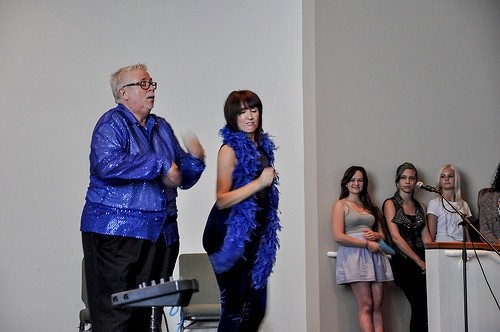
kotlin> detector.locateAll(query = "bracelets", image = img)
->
[366,240,368,248]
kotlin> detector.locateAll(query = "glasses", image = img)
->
[122,81,157,90]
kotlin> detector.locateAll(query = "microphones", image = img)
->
[416,181,436,192]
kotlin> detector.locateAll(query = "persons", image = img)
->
[479,163,500,245]
[202,90,279,332]
[382,162,433,332]
[427,164,472,242]
[333,166,394,332]
[80,65,205,332]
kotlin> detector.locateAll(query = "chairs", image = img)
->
[79,257,91,332]
[179,253,246,332]
